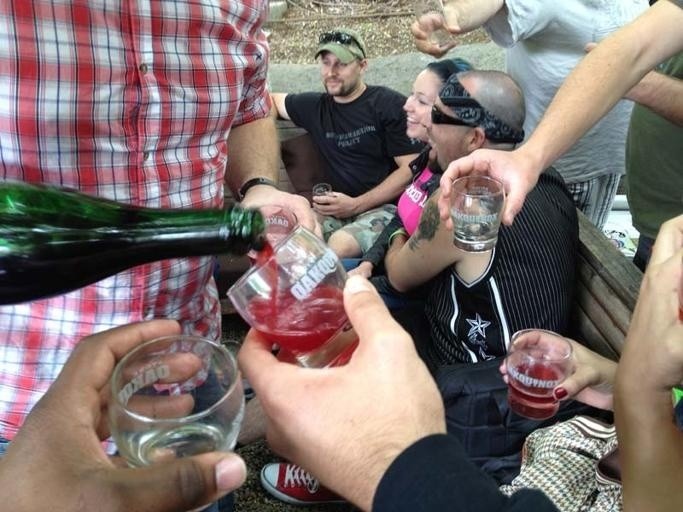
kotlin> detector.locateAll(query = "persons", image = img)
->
[0,0,683,512]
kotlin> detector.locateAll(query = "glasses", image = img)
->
[430,105,479,130]
[317,31,367,59]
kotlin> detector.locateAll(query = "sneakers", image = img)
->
[259,459,355,507]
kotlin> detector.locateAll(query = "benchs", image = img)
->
[214,127,308,316]
[569,202,647,363]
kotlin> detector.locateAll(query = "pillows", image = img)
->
[277,132,351,208]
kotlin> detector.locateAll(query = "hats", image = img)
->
[315,25,367,66]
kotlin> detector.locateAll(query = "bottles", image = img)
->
[0,177,265,308]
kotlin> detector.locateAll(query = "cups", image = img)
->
[504,328,574,420]
[449,175,505,253]
[106,336,246,464]
[242,205,299,259]
[224,223,360,370]
[312,183,334,205]
